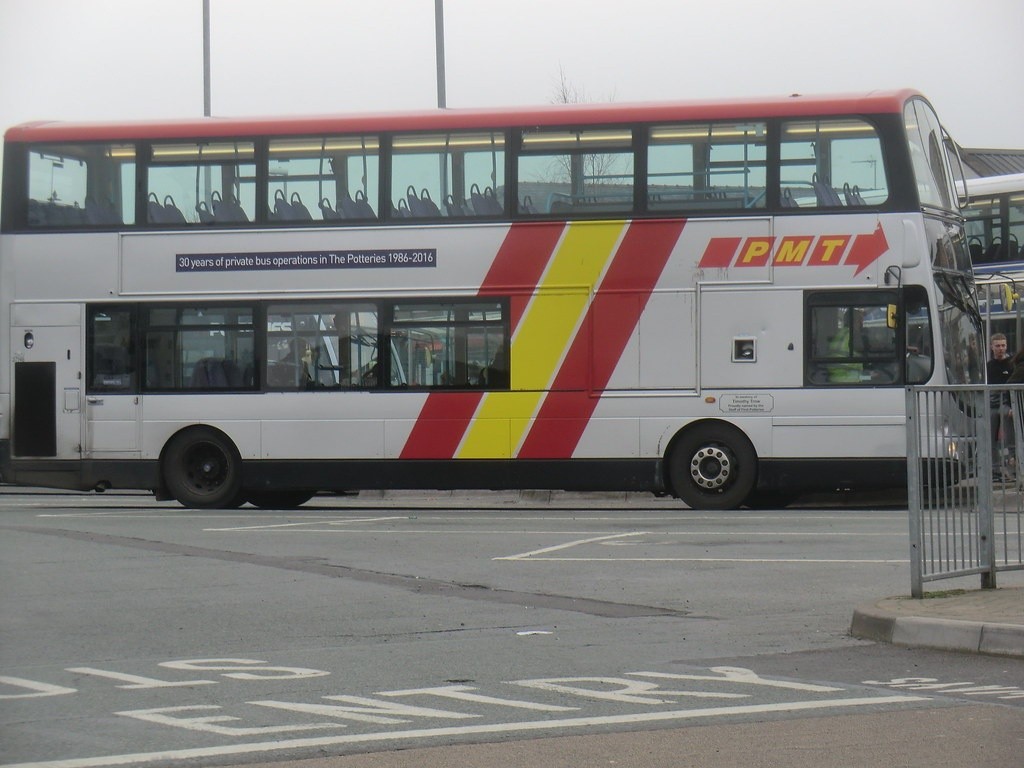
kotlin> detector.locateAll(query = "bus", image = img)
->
[1,89,1024,511]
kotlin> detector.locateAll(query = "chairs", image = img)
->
[99,354,253,393]
[968,231,1023,266]
[23,173,869,233]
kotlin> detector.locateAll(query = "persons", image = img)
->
[988,333,1024,481]
[827,309,888,382]
[945,333,980,384]
[282,338,310,386]
